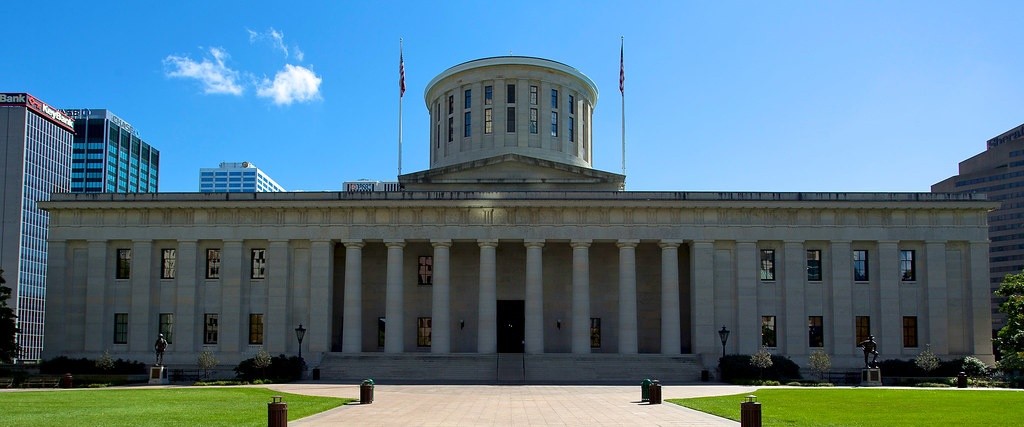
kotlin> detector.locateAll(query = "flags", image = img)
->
[399,49,406,97]
[619,44,625,96]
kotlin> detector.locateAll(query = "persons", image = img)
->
[154,334,168,367]
[860,334,880,369]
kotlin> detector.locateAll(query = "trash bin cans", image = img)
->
[958,372,967,388]
[740,395,762,427]
[64,373,72,388]
[702,370,709,382]
[641,379,652,401]
[368,379,375,401]
[360,380,372,404]
[313,368,320,380]
[649,380,661,404]
[268,396,288,427]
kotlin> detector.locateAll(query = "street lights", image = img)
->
[294,323,307,359]
[718,324,731,357]
